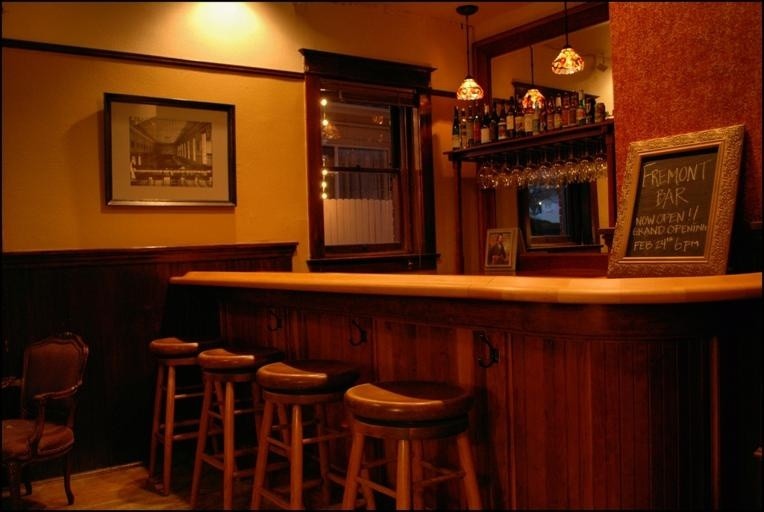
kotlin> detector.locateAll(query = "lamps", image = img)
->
[455,4,484,100]
[551,2,585,76]
[520,45,545,109]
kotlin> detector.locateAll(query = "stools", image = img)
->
[149,337,228,496]
[341,382,484,512]
[190,345,283,511]
[250,359,359,510]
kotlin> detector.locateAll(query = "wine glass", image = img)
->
[479,139,607,192]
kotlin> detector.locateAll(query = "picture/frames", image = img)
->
[607,122,748,278]
[484,227,518,271]
[103,92,237,208]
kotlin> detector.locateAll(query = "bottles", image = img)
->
[489,100,498,142]
[482,103,491,143]
[467,105,473,148]
[506,96,515,138]
[539,99,546,132]
[561,88,570,129]
[515,94,524,137]
[584,97,596,124]
[474,103,481,146]
[459,108,467,149]
[554,94,561,131]
[452,105,461,151]
[576,90,585,126]
[595,103,605,123]
[533,98,539,135]
[545,96,555,130]
[568,89,577,127]
[497,99,508,140]
[525,102,535,134]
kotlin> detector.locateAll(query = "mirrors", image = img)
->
[495,180,608,253]
[490,20,614,120]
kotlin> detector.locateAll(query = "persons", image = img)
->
[487,233,507,264]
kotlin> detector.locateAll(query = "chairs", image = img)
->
[0,331,89,512]
